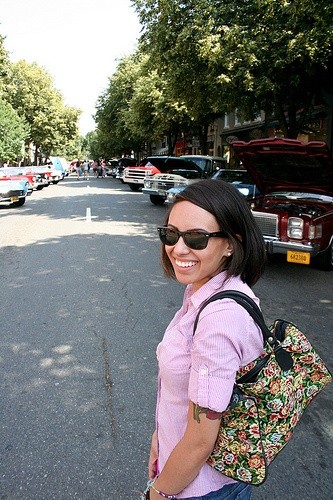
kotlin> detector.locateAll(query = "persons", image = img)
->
[148,179,265,500]
[75,159,106,181]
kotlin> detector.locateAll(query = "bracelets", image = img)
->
[140,475,179,500]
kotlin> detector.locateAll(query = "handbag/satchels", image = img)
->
[190,290,332,487]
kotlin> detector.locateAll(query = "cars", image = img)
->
[229,136,333,271]
[141,154,228,206]
[164,168,260,207]
[0,156,79,207]
[122,155,167,192]
[105,157,138,181]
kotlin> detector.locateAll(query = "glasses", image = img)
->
[157,221,229,251]
